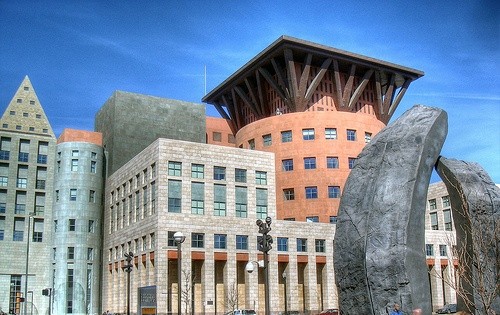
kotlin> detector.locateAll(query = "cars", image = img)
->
[316,308,339,315]
[438,303,457,314]
[225,309,256,315]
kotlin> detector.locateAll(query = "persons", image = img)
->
[389,303,404,315]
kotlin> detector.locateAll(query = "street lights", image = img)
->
[122,251,135,315]
[24,211,35,315]
[173,231,186,315]
[256,217,274,315]
[28,291,33,315]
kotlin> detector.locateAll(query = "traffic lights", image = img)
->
[41,290,48,295]
